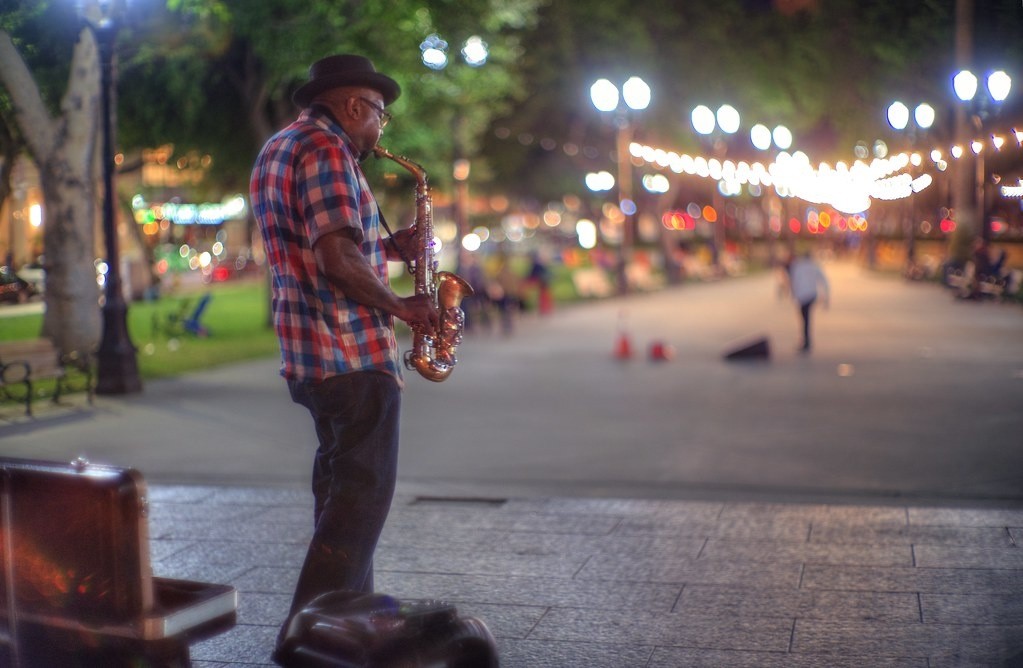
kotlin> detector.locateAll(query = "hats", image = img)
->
[292,55,401,107]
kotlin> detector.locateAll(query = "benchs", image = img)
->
[0,336,94,416]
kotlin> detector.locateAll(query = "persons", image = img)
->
[790,247,831,352]
[958,233,990,300]
[253,76,440,666]
[452,241,555,340]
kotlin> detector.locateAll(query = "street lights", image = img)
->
[748,122,793,264]
[954,70,1011,264]
[886,98,935,261]
[71,0,145,396]
[691,105,741,276]
[591,73,651,292]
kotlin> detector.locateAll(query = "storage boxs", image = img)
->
[0,453,238,668]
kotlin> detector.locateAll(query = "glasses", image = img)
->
[345,97,392,129]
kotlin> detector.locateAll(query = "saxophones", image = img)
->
[369,145,474,382]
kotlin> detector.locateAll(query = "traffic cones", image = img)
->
[725,337,773,359]
[648,342,675,363]
[614,331,634,360]
